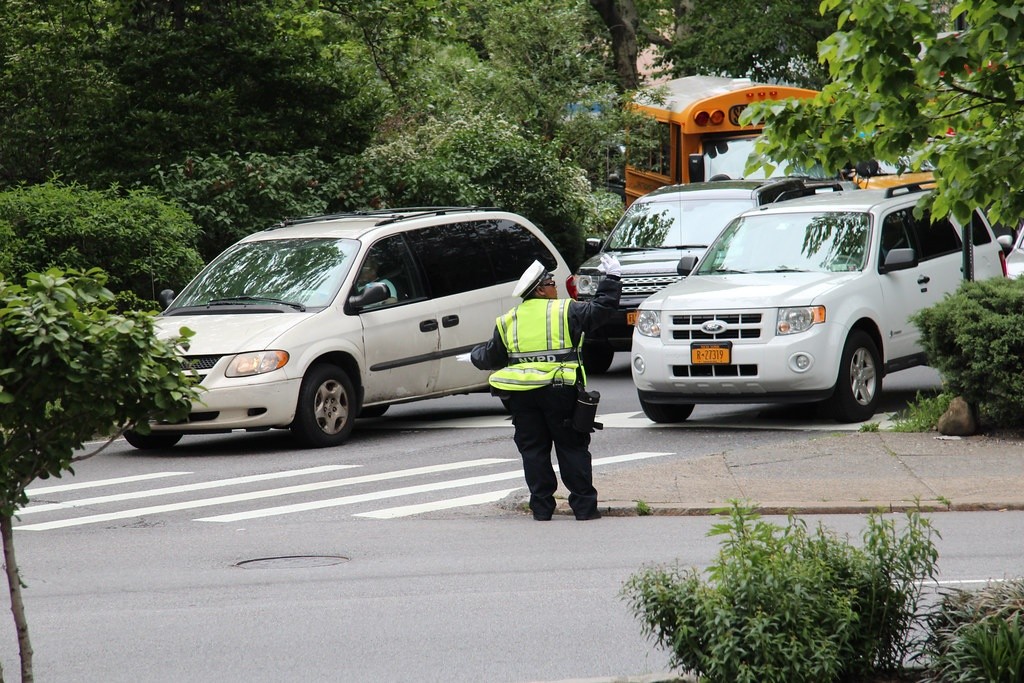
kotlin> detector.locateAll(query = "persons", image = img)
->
[455,252,622,521]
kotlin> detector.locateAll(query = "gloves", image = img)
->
[599,253,622,279]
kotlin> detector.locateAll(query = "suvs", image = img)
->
[629,181,1009,424]
[572,181,815,374]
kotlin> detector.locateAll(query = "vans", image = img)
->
[121,205,579,451]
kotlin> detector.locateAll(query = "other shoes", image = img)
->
[576,509,601,520]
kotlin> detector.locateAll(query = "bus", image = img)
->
[623,74,839,210]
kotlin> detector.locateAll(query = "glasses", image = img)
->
[540,281,556,288]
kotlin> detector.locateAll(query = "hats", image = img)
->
[512,259,555,303]
